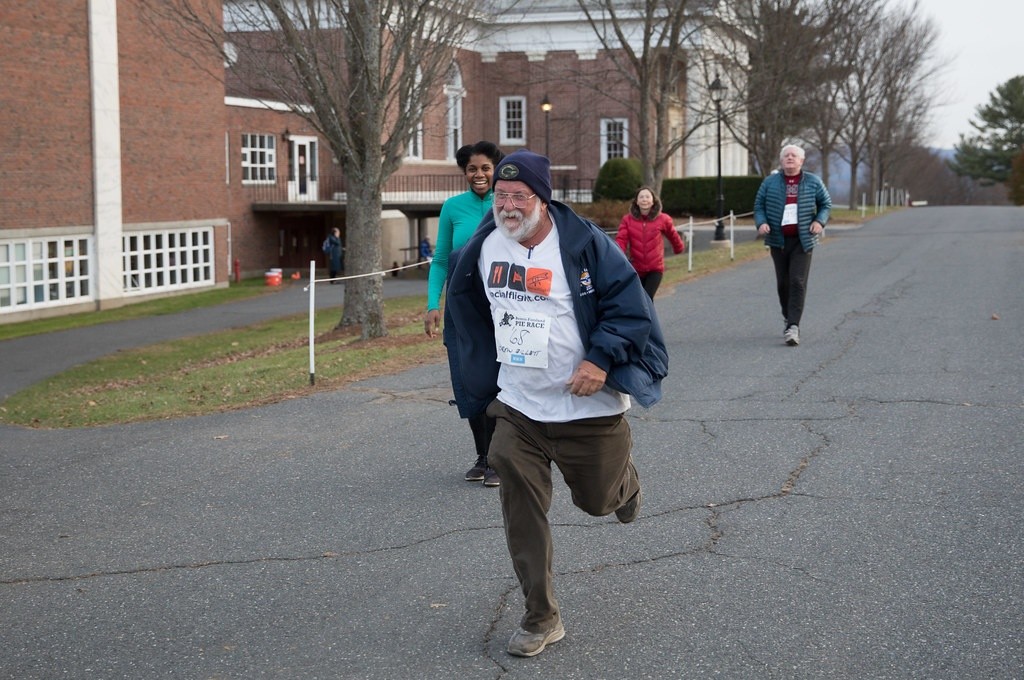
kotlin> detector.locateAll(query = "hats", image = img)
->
[492,148,552,205]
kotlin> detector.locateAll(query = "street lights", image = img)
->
[541,94,555,158]
[709,72,728,240]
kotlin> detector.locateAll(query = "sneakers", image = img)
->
[784,325,800,345]
[615,455,642,523]
[483,463,501,486]
[783,320,788,333]
[466,462,490,480]
[508,617,565,656]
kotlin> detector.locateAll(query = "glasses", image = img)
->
[490,192,536,208]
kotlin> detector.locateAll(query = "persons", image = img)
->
[326,227,344,284]
[754,142,834,346]
[613,184,687,308]
[425,139,509,337]
[443,150,668,659]
[419,236,434,260]
